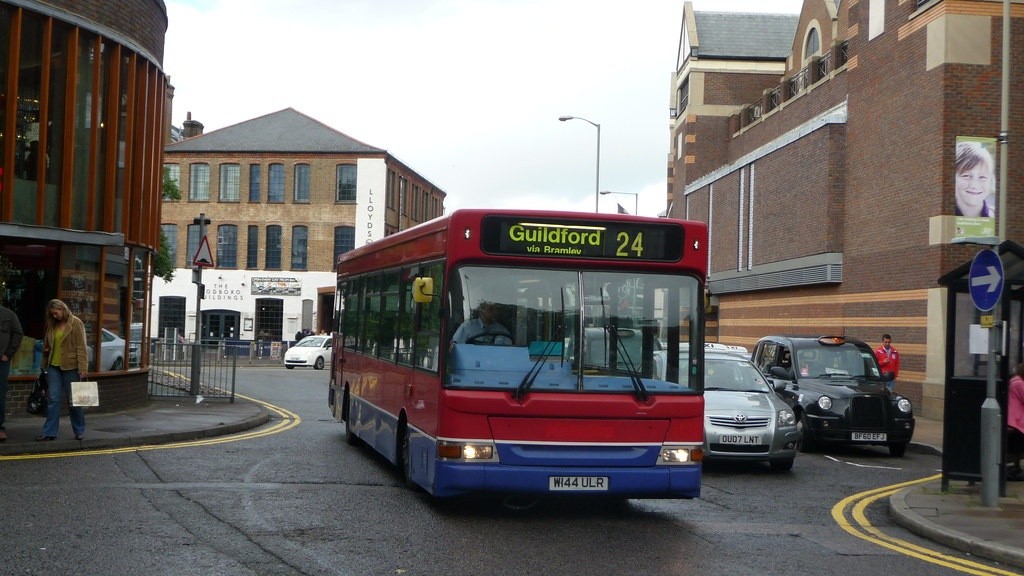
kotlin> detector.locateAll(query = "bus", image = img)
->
[327,209,718,501]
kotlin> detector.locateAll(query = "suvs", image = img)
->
[750,334,916,455]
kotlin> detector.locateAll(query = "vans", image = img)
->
[130,322,143,369]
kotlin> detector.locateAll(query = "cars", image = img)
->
[647,342,800,473]
[83,326,126,372]
[283,336,334,369]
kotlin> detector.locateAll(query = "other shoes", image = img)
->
[0,430,7,440]
[36,435,56,440]
[75,433,84,440]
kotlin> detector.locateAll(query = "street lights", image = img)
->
[558,114,600,213]
[600,190,639,216]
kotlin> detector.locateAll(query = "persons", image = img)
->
[294,328,328,343]
[955,141,996,217]
[874,334,899,392]
[1007,363,1024,481]
[447,296,512,352]
[34,299,89,441]
[1,304,23,441]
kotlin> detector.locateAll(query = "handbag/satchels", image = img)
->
[25,379,47,416]
[70,375,99,407]
